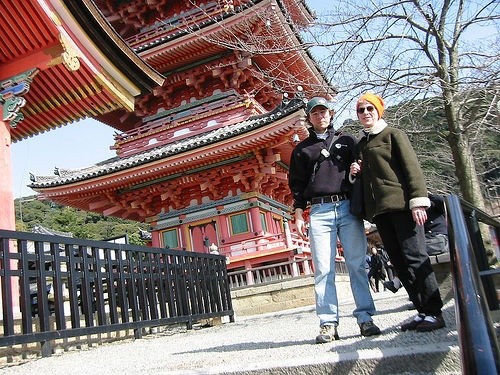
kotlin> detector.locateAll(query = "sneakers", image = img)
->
[360,322,380,336]
[316,324,339,343]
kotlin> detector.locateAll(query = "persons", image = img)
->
[289,97,381,344]
[345,92,446,332]
[381,191,448,293]
[363,246,394,293]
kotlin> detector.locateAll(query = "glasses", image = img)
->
[358,106,376,114]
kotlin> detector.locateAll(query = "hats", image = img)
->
[307,97,330,113]
[358,93,385,119]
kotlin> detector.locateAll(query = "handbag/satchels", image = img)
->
[349,177,365,218]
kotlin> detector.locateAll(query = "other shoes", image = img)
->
[401,314,426,331]
[384,281,398,293]
[417,316,446,331]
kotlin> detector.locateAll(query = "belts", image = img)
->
[312,194,351,204]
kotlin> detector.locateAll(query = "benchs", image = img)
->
[429,252,454,303]
